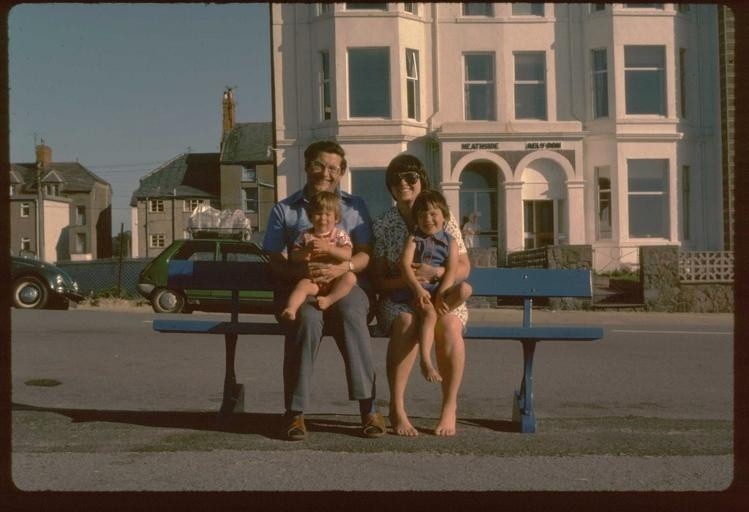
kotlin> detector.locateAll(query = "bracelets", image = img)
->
[435,266,439,282]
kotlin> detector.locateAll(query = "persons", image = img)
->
[259,140,386,440]
[364,154,473,437]
[400,189,473,384]
[281,190,358,322]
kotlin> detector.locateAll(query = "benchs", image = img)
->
[152,261,603,432]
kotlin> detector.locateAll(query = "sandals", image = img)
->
[281,411,308,440]
[360,410,386,438]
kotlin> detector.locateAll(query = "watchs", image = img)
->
[348,260,355,272]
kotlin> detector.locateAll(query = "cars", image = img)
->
[9,250,86,312]
[131,224,293,318]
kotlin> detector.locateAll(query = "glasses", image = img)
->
[307,160,342,176]
[389,172,422,186]
